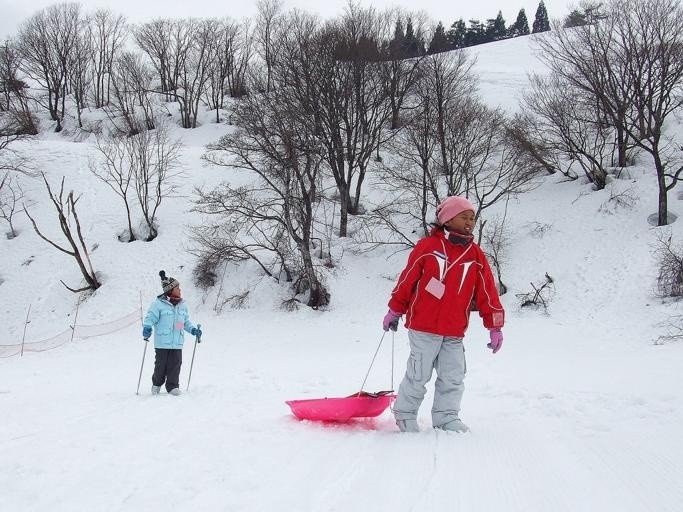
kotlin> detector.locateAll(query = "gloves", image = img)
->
[383,308,402,331]
[487,328,503,353]
[143,327,152,338]
[192,328,202,336]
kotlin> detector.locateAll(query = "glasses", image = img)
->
[444,228,473,245]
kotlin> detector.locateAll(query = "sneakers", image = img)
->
[152,385,161,394]
[396,419,420,433]
[442,419,469,433]
[169,388,182,396]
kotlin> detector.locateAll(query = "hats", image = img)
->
[159,270,179,293]
[436,196,475,224]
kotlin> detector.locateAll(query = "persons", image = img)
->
[382,195,505,435]
[142,269,202,395]
